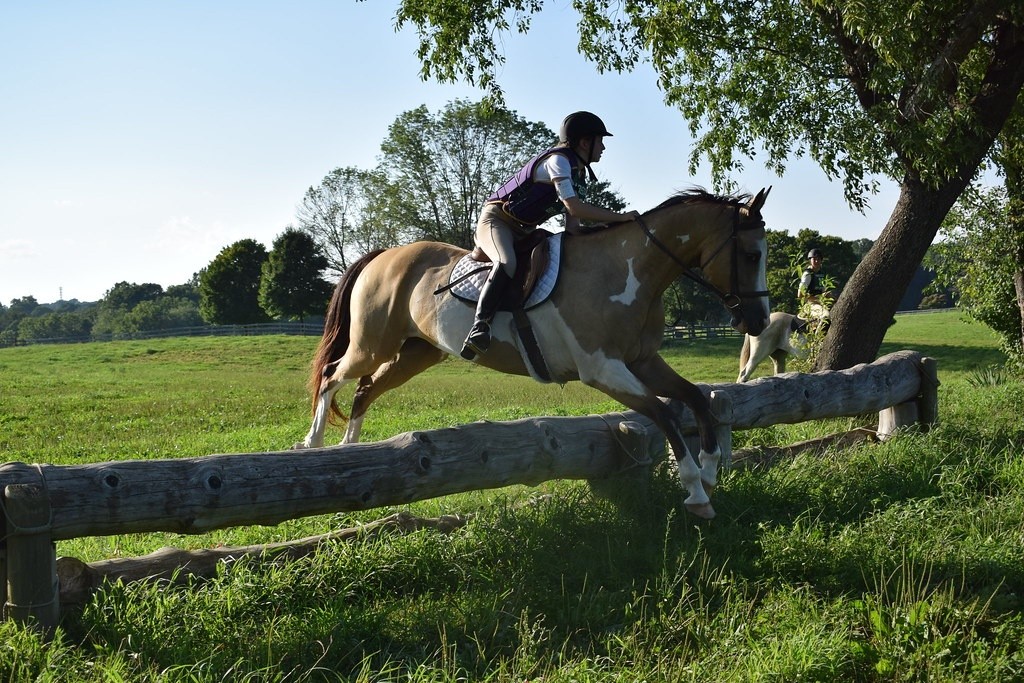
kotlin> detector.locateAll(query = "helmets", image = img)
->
[559,111,613,142]
[808,248,823,259]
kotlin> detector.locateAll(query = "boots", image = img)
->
[459,261,512,361]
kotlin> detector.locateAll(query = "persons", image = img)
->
[460,112,640,360]
[798,249,829,304]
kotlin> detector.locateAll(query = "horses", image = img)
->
[736,311,830,383]
[294,186,775,521]
